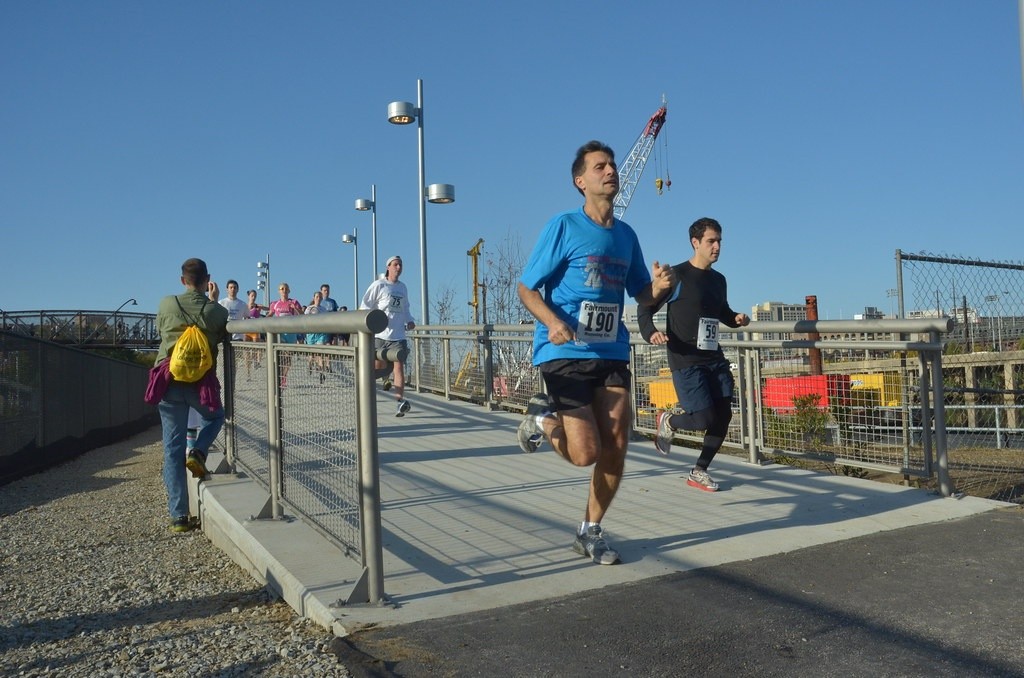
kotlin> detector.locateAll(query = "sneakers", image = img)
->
[383,376,392,391]
[169,516,188,532]
[573,522,618,564]
[396,401,410,417]
[654,410,675,455]
[185,449,210,477]
[517,393,550,454]
[687,467,720,492]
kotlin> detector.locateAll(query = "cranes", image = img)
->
[492,93,672,408]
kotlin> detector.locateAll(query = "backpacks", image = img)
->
[170,295,212,383]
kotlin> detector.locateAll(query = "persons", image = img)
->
[266,282,304,388]
[218,280,249,342]
[302,292,328,384]
[144,258,229,532]
[320,284,339,373]
[339,306,350,347]
[516,140,675,565]
[637,217,751,493]
[243,290,261,382]
[0,321,109,343]
[117,322,157,343]
[358,255,416,417]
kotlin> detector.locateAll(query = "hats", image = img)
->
[385,256,402,277]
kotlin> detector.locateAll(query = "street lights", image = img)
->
[355,183,377,284]
[387,80,455,389]
[257,253,269,307]
[342,227,359,311]
